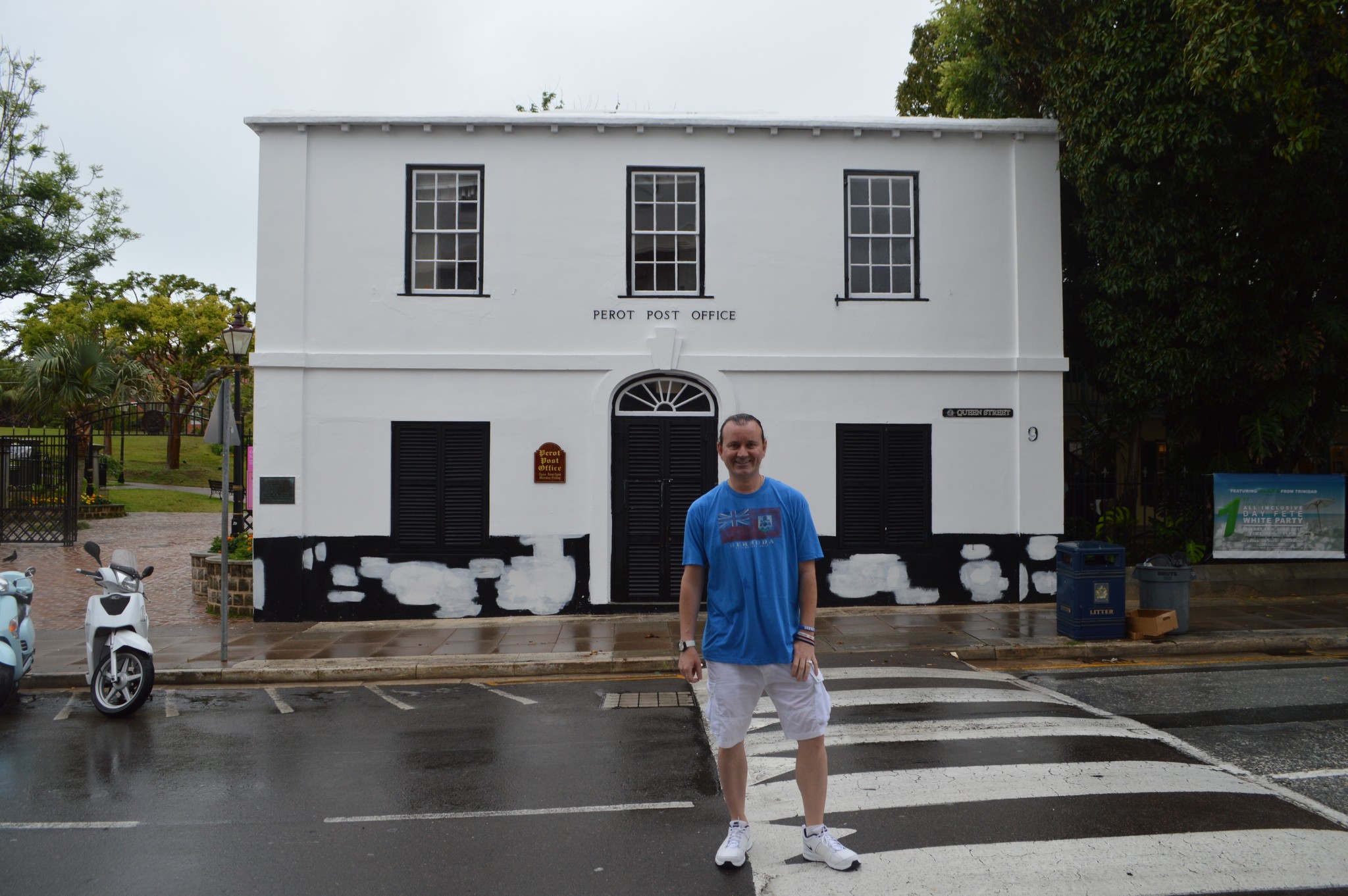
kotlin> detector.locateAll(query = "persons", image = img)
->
[678,413,862,872]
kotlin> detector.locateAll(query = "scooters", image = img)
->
[0,567,36,707]
[75,541,155,718]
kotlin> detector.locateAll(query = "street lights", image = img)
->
[118,397,131,483]
[221,303,255,552]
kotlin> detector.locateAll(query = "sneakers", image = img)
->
[801,824,861,871]
[715,816,752,870]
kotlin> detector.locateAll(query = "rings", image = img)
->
[806,660,813,665]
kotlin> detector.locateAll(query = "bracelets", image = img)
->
[795,631,815,646]
[798,625,815,632]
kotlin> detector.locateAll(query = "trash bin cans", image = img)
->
[1055,540,1126,640]
[1131,563,1197,635]
[0,437,44,486]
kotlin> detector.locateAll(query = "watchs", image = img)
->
[677,640,696,651]
